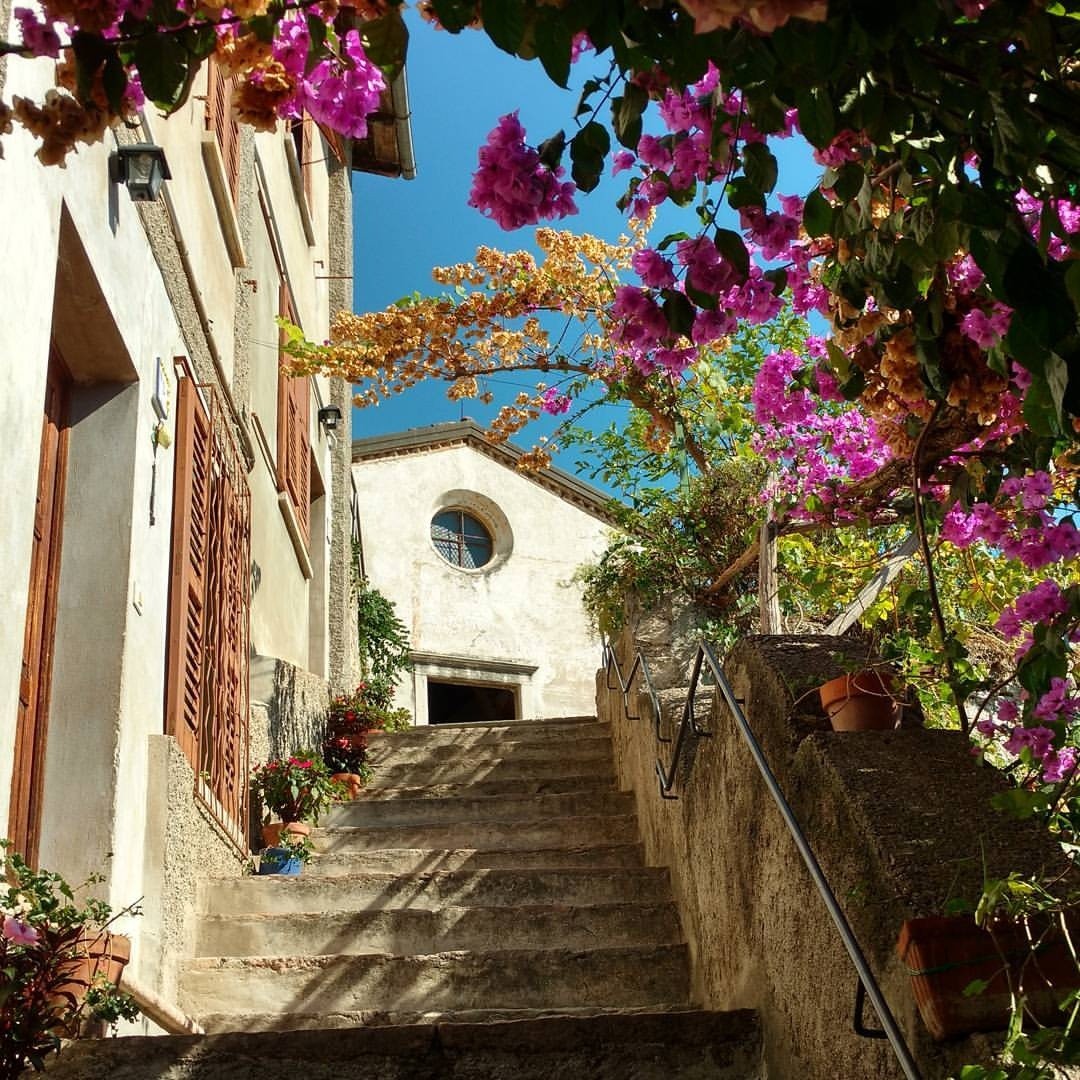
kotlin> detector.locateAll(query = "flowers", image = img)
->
[0,837,145,1080]
[325,697,374,732]
[321,737,376,789]
[251,750,337,823]
[367,703,412,731]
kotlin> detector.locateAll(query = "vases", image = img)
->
[333,729,370,750]
[34,930,132,1040]
[369,718,384,729]
[329,769,362,801]
[262,822,310,848]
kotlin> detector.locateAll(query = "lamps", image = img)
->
[317,405,342,430]
[106,140,173,201]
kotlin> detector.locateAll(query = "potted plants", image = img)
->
[822,612,920,733]
[894,871,1080,1045]
[260,829,317,874]
